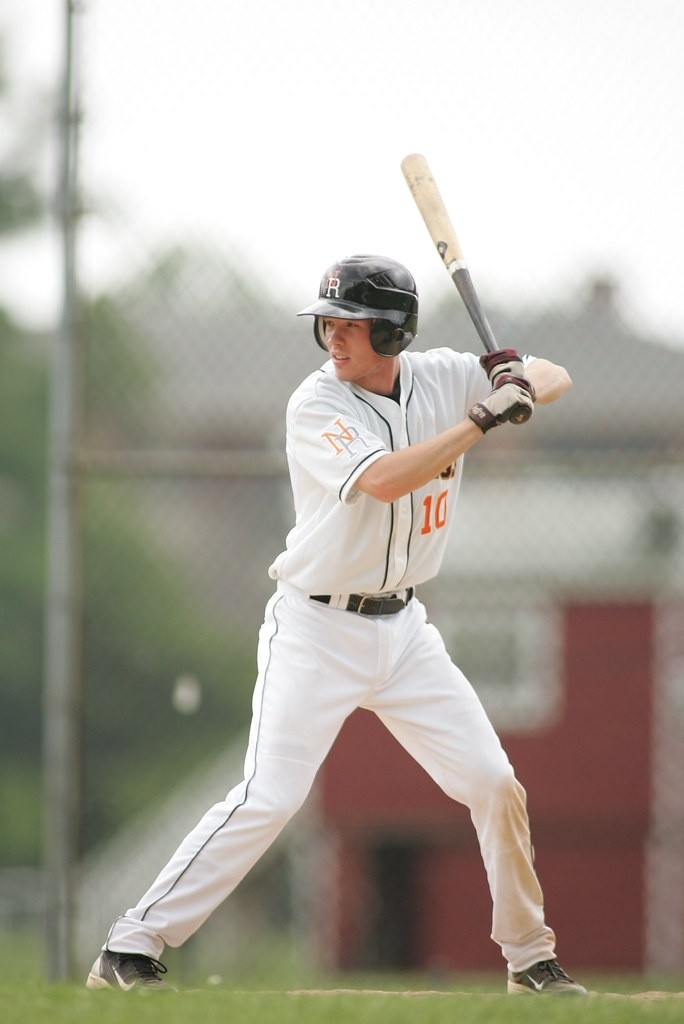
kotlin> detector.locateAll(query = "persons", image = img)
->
[85,254,591,998]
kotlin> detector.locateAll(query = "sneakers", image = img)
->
[85,948,179,996]
[506,959,587,998]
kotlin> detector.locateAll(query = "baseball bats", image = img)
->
[400,151,531,425]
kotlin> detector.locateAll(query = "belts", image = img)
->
[310,585,413,618]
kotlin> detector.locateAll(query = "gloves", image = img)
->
[465,374,536,434]
[480,349,524,390]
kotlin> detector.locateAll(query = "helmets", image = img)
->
[297,254,419,357]
